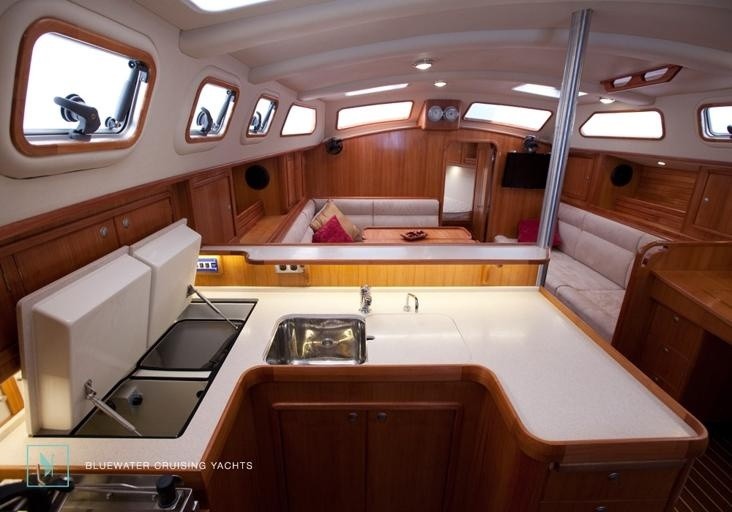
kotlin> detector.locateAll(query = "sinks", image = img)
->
[262,314,367,365]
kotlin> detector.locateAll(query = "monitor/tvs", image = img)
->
[501,152,551,189]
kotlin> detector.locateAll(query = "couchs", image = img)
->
[494,203,669,344]
[267,197,440,248]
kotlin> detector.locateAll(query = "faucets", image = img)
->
[361,284,372,313]
[407,293,419,313]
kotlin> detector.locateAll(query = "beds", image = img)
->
[361,226,471,241]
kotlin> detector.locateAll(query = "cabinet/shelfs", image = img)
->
[485,399,705,509]
[249,380,484,511]
[636,298,727,421]
[0,183,187,352]
[182,173,242,244]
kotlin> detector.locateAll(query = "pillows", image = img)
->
[511,216,562,247]
[309,198,365,244]
